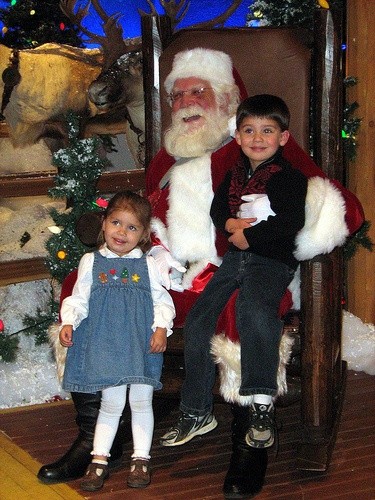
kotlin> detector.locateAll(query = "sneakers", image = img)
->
[160,411,218,446]
[245,401,275,448]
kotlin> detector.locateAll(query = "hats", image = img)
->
[164,46,248,136]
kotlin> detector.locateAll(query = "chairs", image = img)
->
[69,15,358,473]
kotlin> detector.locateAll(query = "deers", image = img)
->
[60,0,243,131]
[0,41,146,166]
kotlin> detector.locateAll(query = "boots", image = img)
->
[223,401,267,497]
[35,391,123,482]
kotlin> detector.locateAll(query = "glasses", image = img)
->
[171,87,213,101]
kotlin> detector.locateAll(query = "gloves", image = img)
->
[149,245,186,292]
[234,192,276,227]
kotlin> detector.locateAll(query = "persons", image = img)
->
[161,94,308,448]
[59,191,175,491]
[38,47,364,500]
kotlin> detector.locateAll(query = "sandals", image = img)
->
[128,460,152,487]
[80,462,110,491]
